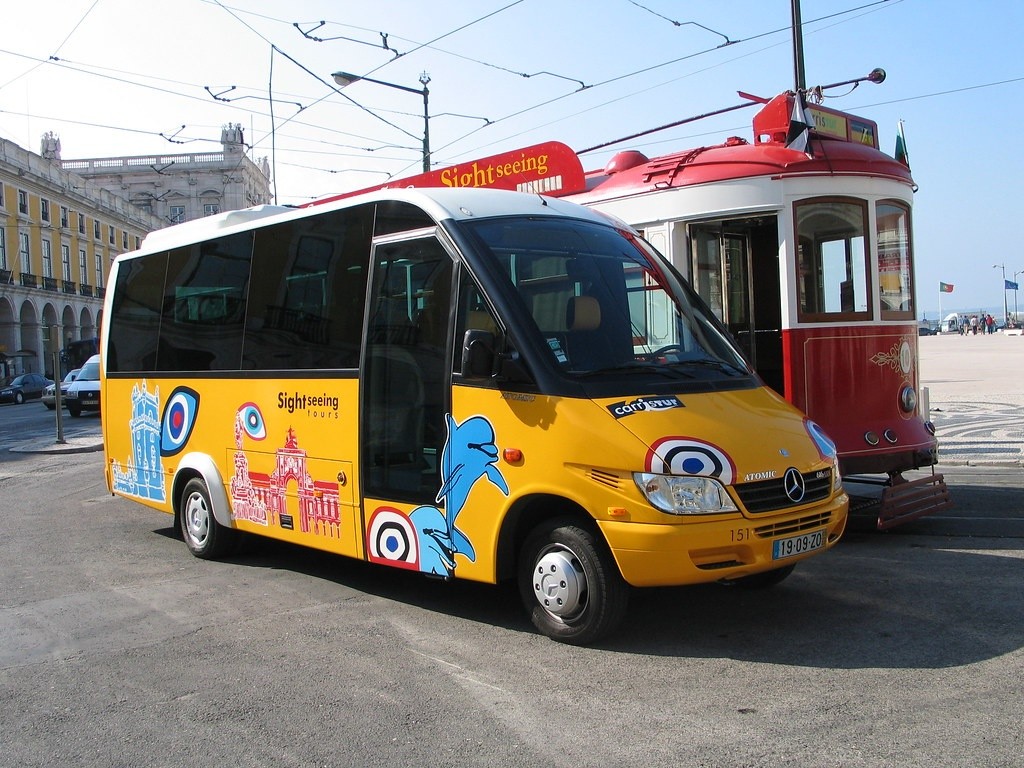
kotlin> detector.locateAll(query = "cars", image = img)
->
[918,327,937,336]
[42,368,80,409]
[0,373,55,406]
[992,319,1023,332]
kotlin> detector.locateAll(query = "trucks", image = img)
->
[941,311,986,332]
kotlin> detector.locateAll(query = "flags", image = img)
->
[1005,280,1018,290]
[940,282,954,293]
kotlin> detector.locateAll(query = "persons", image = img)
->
[970,315,979,335]
[986,315,992,334]
[992,317,997,331]
[980,314,986,335]
[957,315,970,336]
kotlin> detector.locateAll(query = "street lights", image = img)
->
[66,331,73,344]
[333,72,431,173]
[992,264,1007,326]
[1014,270,1024,322]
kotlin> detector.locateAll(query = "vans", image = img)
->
[65,354,102,418]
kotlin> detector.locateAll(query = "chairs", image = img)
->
[564,296,614,371]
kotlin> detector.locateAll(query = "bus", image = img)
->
[98,186,849,644]
[302,89,956,530]
